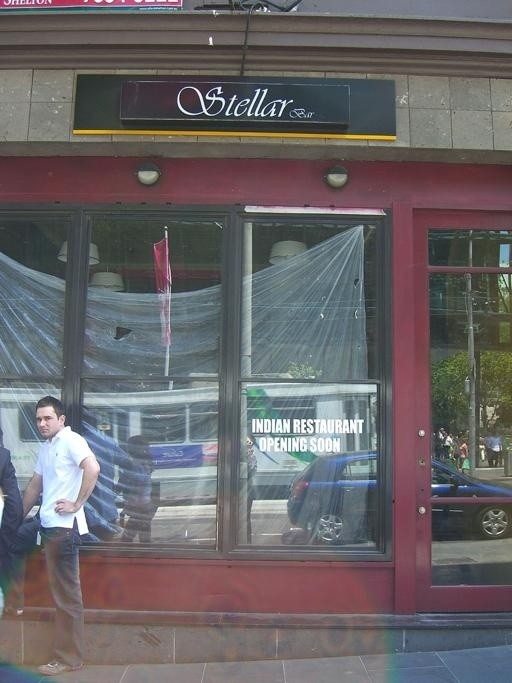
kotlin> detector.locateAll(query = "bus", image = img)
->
[0,370,382,499]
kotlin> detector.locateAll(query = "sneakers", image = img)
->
[38,660,84,675]
[3,606,23,617]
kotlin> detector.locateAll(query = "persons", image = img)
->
[430,427,504,473]
[14,395,102,678]
[246,435,258,541]
[0,432,23,618]
[71,403,160,543]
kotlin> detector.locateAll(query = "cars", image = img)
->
[287,451,512,543]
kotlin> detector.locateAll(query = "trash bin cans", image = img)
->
[503,447,512,477]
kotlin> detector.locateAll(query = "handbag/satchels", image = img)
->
[124,482,161,519]
